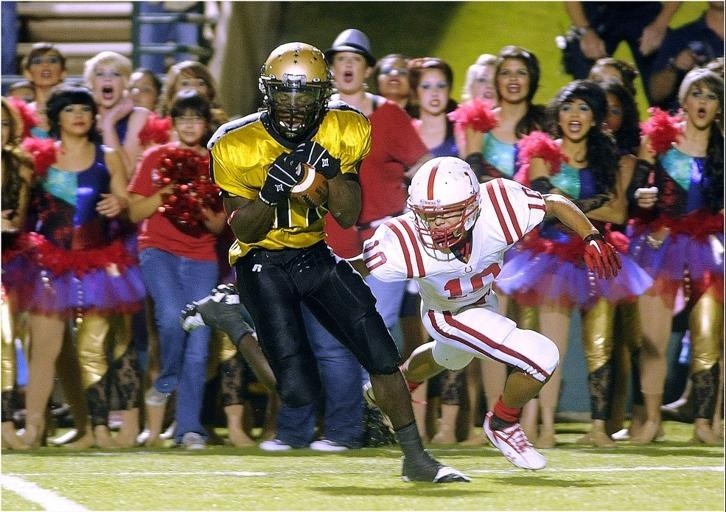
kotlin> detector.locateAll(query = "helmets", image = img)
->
[408,157,481,249]
[259,42,331,138]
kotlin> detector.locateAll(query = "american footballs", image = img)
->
[287,164,329,208]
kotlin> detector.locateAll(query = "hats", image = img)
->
[325,30,376,69]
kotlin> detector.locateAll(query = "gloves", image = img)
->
[584,235,622,280]
[291,142,341,178]
[259,153,302,206]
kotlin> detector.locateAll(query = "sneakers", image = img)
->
[261,437,292,450]
[362,383,397,435]
[403,454,468,484]
[144,385,168,404]
[181,284,238,332]
[483,413,550,470]
[182,432,207,449]
[311,439,350,451]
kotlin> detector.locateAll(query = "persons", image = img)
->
[13,91,132,451]
[358,156,621,470]
[125,65,158,136]
[557,1,681,86]
[594,86,638,441]
[83,52,151,428]
[411,60,486,446]
[459,51,516,138]
[477,47,553,448]
[632,71,724,447]
[371,50,429,447]
[514,82,625,459]
[210,40,466,482]
[648,3,721,106]
[134,61,229,447]
[128,100,233,451]
[305,28,432,445]
[1,92,31,451]
[581,56,638,85]
[9,48,70,141]
